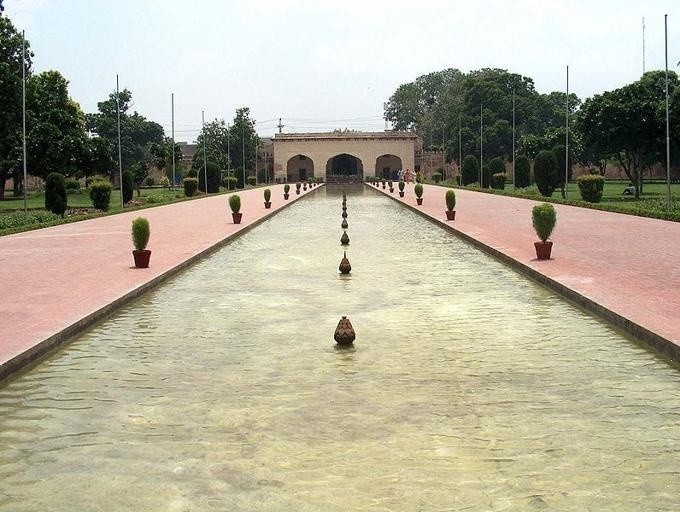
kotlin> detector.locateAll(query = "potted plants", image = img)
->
[129,217,152,267]
[227,175,324,223]
[529,201,560,263]
[365,175,457,221]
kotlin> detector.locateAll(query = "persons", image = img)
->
[621,183,635,195]
[391,168,421,184]
